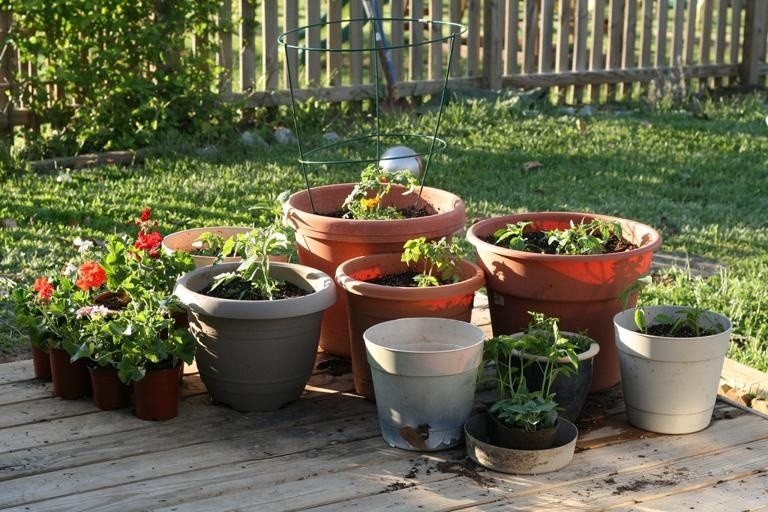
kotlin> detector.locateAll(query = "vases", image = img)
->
[28,326,181,421]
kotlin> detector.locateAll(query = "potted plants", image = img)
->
[282,163,465,357]
[464,312,602,476]
[612,276,733,436]
[336,235,486,399]
[464,210,663,397]
[159,191,337,416]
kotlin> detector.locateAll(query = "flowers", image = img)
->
[24,218,196,380]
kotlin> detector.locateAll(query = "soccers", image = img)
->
[377,146,424,180]
